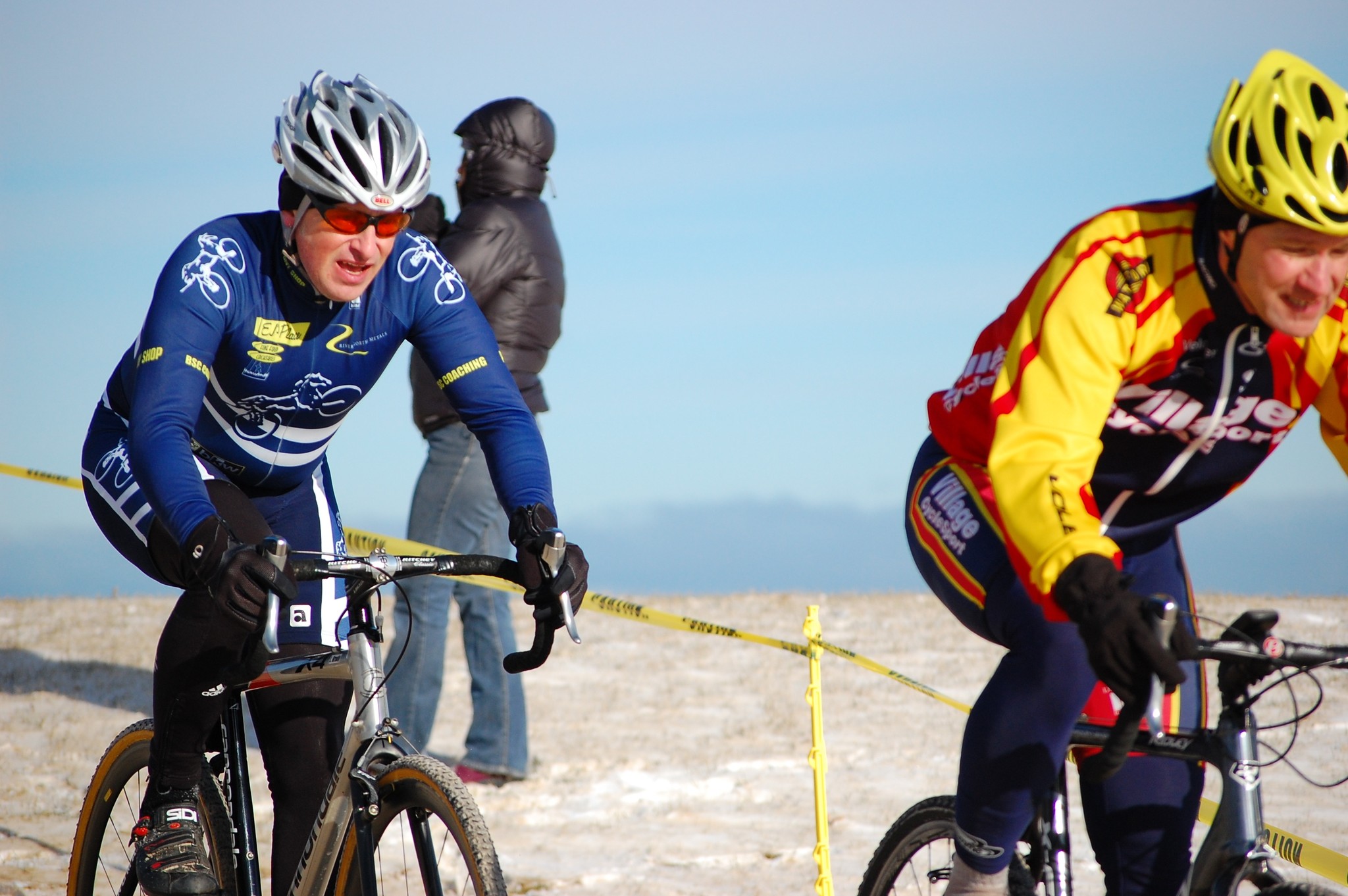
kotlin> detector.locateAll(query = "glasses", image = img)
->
[312,194,415,239]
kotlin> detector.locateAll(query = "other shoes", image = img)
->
[450,766,524,788]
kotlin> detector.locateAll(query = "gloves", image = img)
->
[506,503,589,631]
[1052,554,1189,702]
[183,516,298,629]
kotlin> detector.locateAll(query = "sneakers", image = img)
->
[125,797,217,894]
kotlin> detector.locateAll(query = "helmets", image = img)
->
[1202,47,1348,238]
[272,67,433,210]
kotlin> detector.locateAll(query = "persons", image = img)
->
[86,68,592,896]
[372,99,566,782]
[906,45,1347,896]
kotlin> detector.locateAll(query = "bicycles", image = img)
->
[858,589,1348,896]
[66,525,584,896]
